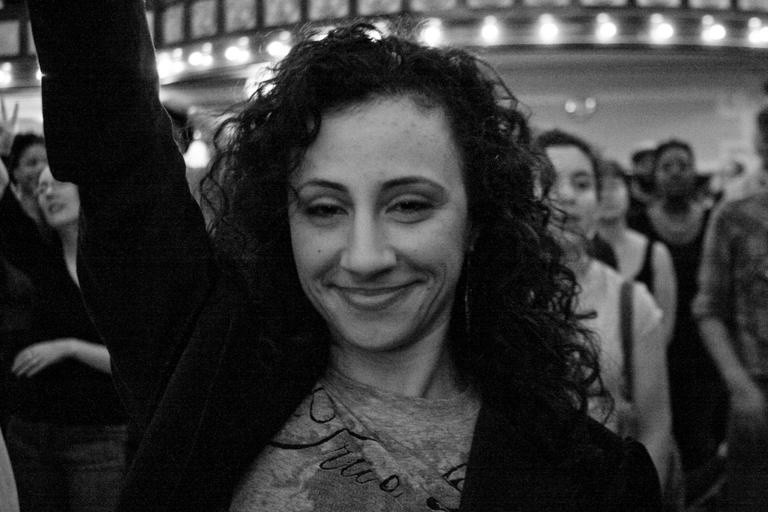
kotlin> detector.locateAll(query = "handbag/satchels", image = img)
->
[613,402,687,510]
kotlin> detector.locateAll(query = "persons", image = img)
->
[1,98,195,510]
[27,0,668,511]
[531,104,768,511]
